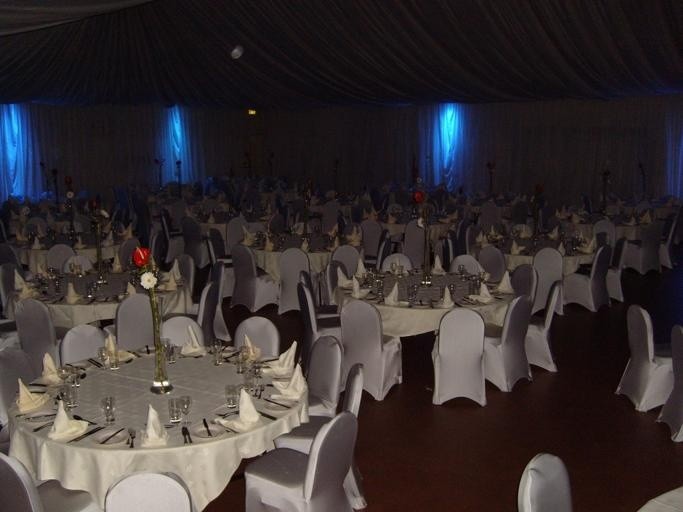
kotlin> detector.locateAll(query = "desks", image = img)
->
[5,345,312,512]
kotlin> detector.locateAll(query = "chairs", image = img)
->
[0,451,42,512]
[241,410,359,512]
[273,363,367,512]
[105,470,192,512]
[516,451,573,511]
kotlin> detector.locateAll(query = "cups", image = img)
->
[27,200,160,298]
[190,198,683,255]
[361,261,481,300]
[57,338,261,426]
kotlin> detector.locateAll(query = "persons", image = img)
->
[206,173,259,212]
[581,192,592,214]
[414,176,425,193]
[661,204,683,327]
[456,187,464,196]
[4,198,32,226]
[321,191,338,225]
[435,184,447,192]
[287,176,320,208]
[170,201,207,255]
[101,184,149,234]
[530,183,544,225]
[72,198,95,240]
[364,184,389,209]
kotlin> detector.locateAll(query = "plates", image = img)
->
[457,302,479,307]
[15,345,293,447]
[412,303,431,308]
